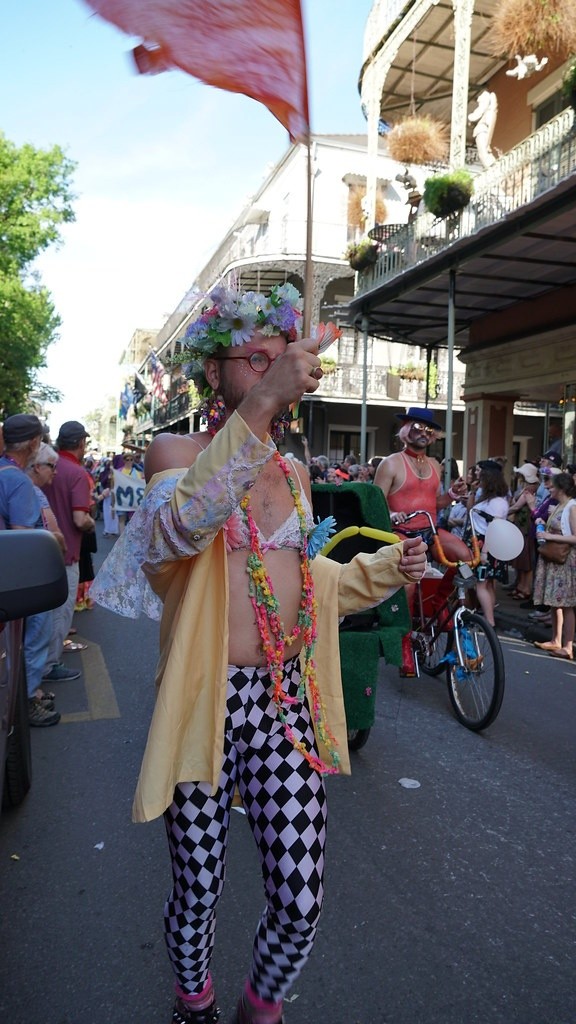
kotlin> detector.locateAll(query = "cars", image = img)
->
[0,520,70,810]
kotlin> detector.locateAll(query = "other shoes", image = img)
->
[550,651,573,660]
[507,588,554,626]
[235,980,285,1024]
[26,697,61,726]
[62,641,87,652]
[40,692,55,700]
[40,666,82,681]
[535,642,560,651]
[172,972,221,1024]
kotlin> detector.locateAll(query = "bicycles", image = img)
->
[392,506,512,733]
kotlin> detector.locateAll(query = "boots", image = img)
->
[430,570,458,631]
[399,598,414,674]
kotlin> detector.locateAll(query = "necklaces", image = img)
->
[186,438,342,775]
[403,449,425,463]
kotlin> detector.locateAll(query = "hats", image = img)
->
[60,421,90,443]
[539,451,562,468]
[524,459,540,467]
[335,468,349,480]
[540,468,562,478]
[3,414,42,441]
[395,408,443,430]
[513,463,540,483]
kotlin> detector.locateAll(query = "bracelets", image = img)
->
[448,488,461,500]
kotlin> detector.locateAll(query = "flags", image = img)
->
[82,0,308,146]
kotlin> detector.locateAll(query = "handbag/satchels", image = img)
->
[537,538,571,564]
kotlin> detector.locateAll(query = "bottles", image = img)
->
[536,521,546,545]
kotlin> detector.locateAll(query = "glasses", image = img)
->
[410,422,434,435]
[31,462,57,471]
[126,459,133,461]
[223,351,284,373]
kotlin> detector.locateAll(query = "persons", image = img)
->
[83,452,145,608]
[0,414,88,726]
[441,451,576,658]
[130,284,427,1024]
[372,407,467,678]
[301,436,383,484]
